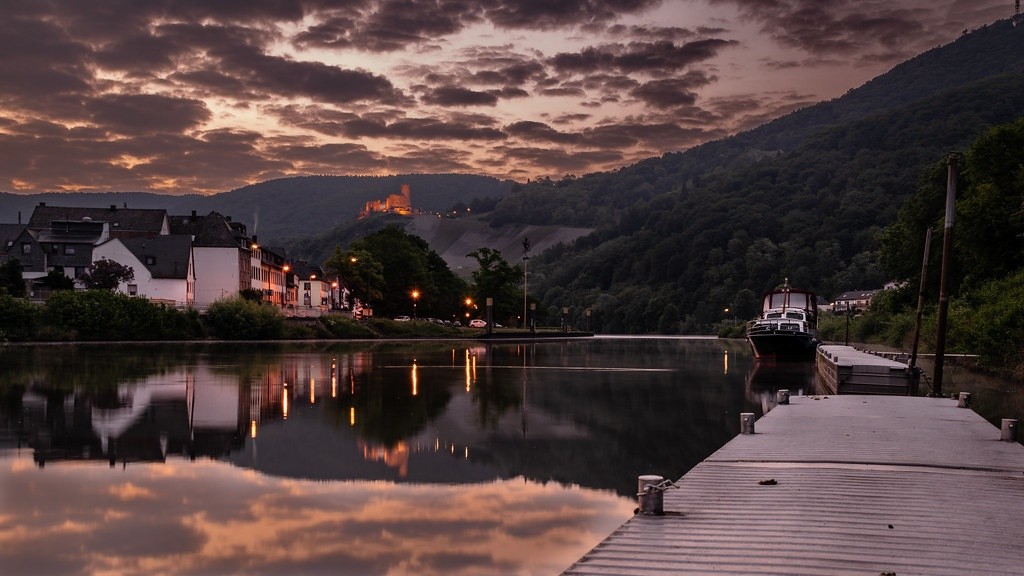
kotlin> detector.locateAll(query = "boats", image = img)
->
[745,277,820,360]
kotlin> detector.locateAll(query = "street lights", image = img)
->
[281,266,289,310]
[466,298,472,313]
[351,257,371,320]
[309,274,316,309]
[412,291,420,321]
[330,283,337,309]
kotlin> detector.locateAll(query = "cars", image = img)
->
[418,317,452,327]
[469,319,488,328]
[394,315,410,323]
[453,321,461,327]
[492,322,503,328]
